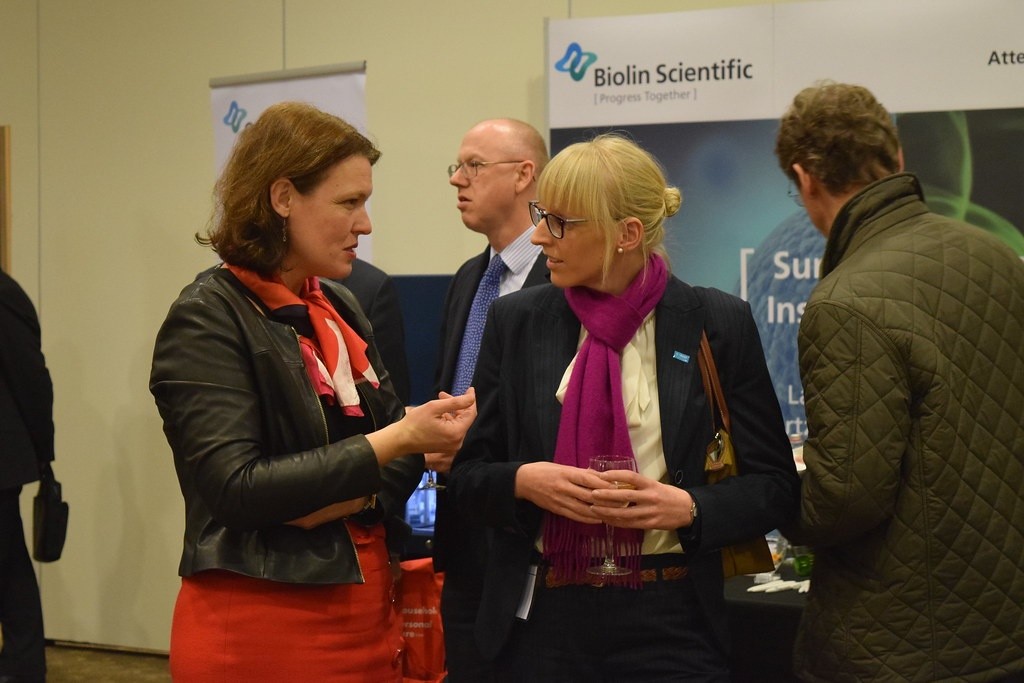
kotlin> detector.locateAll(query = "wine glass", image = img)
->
[587,456,635,575]
[411,405,457,490]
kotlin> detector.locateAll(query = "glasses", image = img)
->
[529,200,619,237]
[786,176,805,209]
[449,159,538,183]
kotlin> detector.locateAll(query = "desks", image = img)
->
[722,577,807,683]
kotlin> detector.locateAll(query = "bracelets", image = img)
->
[690,500,697,525]
[347,493,376,520]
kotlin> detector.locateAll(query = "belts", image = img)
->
[544,565,687,588]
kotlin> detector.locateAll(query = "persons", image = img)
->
[324,259,424,583]
[445,134,799,683]
[775,85,1023,683]
[149,101,478,683]
[433,117,552,683]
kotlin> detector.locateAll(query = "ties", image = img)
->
[452,253,505,398]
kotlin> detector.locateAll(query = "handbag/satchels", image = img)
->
[694,333,776,579]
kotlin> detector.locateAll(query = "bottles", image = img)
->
[789,433,807,472]
